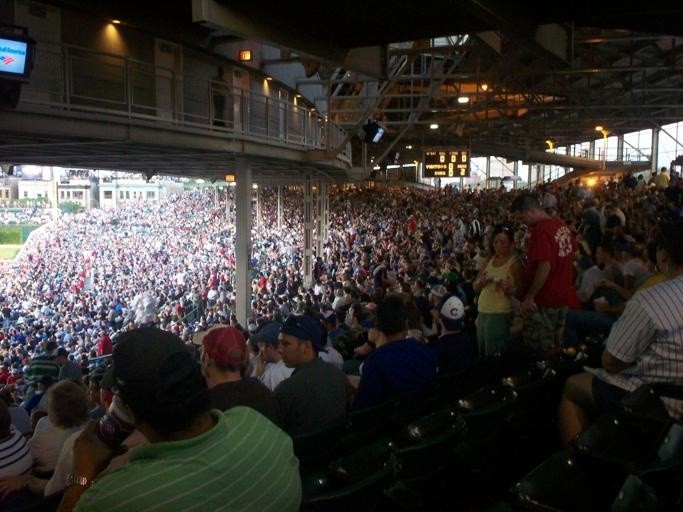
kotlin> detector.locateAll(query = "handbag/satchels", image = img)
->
[616,385,672,460]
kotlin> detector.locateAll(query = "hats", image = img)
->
[99,328,191,393]
[193,325,248,364]
[249,323,281,345]
[431,285,447,296]
[436,293,465,319]
[278,314,322,344]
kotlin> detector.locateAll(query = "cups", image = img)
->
[87,394,135,450]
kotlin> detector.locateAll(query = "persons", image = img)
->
[207,64,233,127]
[0,23,38,114]
[350,132,362,162]
[1,152,683,512]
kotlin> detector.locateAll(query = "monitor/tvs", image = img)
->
[389,150,400,164]
[0,34,37,85]
[362,122,385,144]
